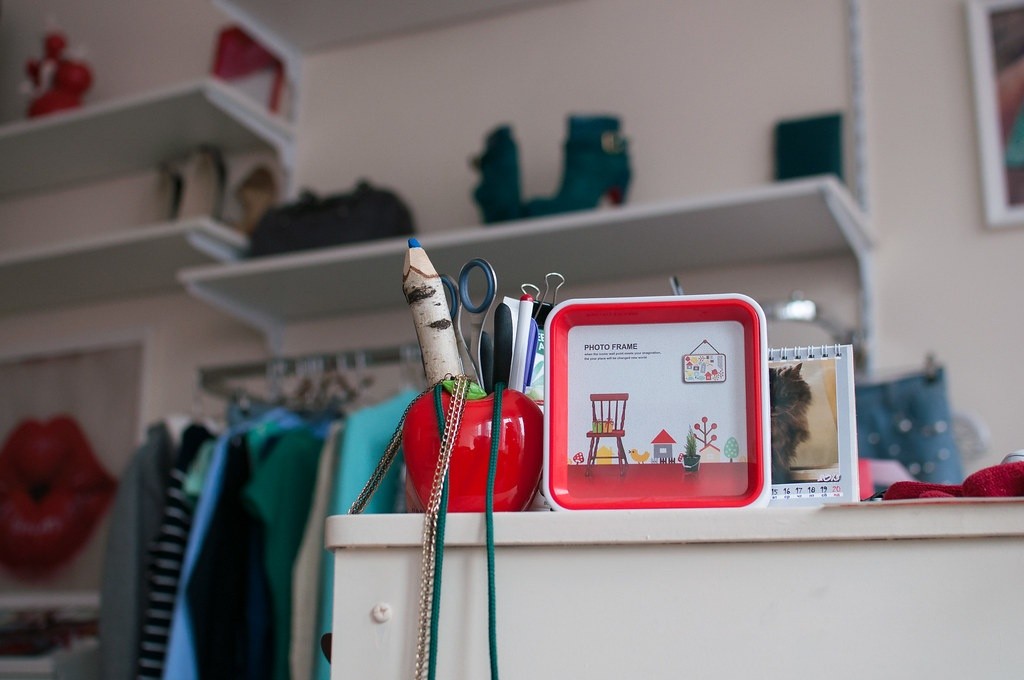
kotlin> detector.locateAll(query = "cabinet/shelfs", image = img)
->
[176,172,880,406]
[0,0,306,311]
[324,503,1024,680]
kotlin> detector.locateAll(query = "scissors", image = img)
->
[437,257,498,400]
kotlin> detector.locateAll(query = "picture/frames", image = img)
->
[962,0,1024,229]
[542,292,772,511]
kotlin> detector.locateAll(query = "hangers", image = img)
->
[834,326,947,403]
[181,337,426,422]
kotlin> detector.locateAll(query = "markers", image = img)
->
[399,238,540,395]
